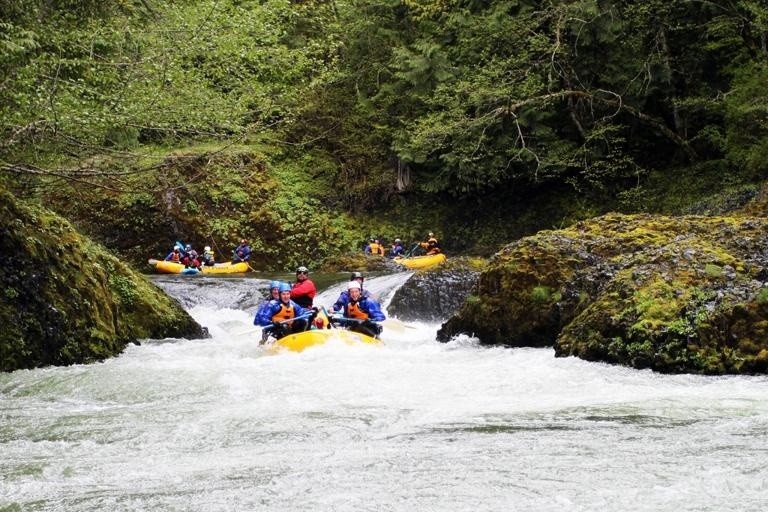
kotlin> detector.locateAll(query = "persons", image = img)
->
[164,239,252,271]
[365,232,438,259]
[253,266,386,340]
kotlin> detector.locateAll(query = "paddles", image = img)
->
[240,310,316,335]
[334,317,416,330]
[176,241,186,252]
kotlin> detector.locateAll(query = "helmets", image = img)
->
[296,266,309,279]
[375,240,380,244]
[369,238,374,243]
[269,281,280,293]
[348,281,361,296]
[427,232,435,239]
[174,244,211,261]
[278,283,291,293]
[351,272,363,282]
[395,238,402,246]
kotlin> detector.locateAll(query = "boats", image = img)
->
[147,258,249,274]
[393,253,446,269]
[267,329,386,356]
